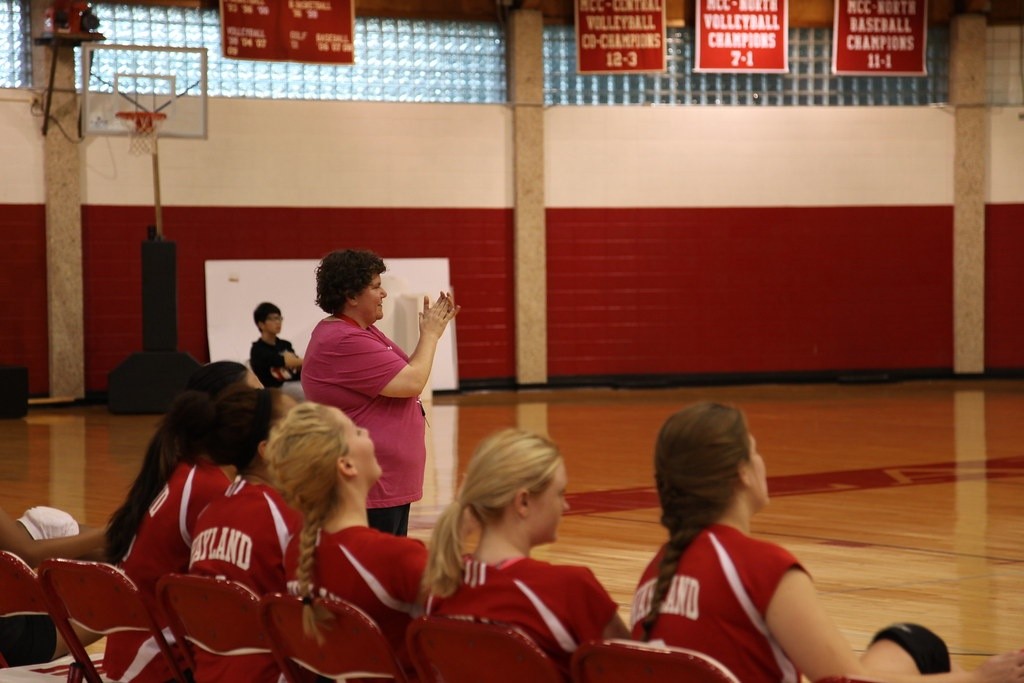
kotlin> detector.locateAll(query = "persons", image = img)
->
[630,401,1024,683]
[265,401,429,683]
[103,361,265,683]
[300,248,460,536]
[157,382,304,683]
[248,301,304,387]
[419,431,630,683]
[0,500,137,683]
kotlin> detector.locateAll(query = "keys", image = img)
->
[421,404,431,428]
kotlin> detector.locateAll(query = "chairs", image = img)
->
[0,548,739,683]
